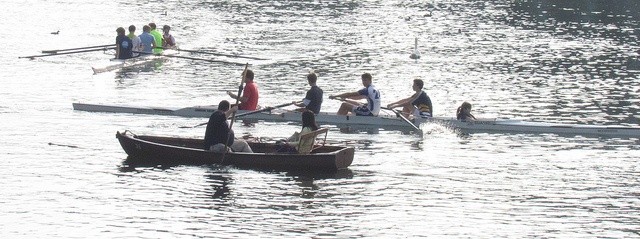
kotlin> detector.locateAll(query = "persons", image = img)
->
[115,27,133,59]
[276,110,318,154]
[387,79,433,119]
[127,25,144,57]
[456,102,476,121]
[287,73,323,114]
[204,100,254,154]
[138,25,156,55]
[149,23,167,52]
[335,73,381,117]
[229,70,259,110]
[161,25,175,50]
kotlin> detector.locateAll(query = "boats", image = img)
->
[115,129,356,172]
[91,48,178,75]
[71,100,640,138]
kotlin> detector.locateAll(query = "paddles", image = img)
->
[391,107,424,138]
[179,99,303,129]
[41,42,118,54]
